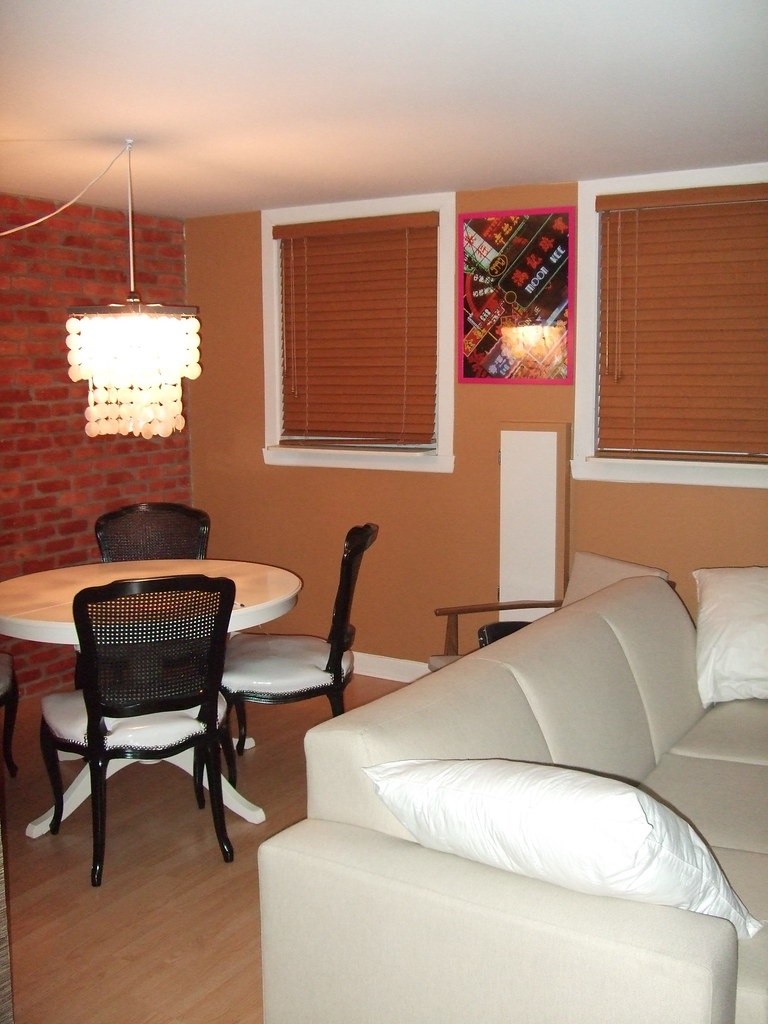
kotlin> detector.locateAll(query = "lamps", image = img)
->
[65,139,201,439]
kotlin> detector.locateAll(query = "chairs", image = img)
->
[429,552,669,672]
[1,651,19,780]
[40,573,236,888]
[218,523,379,784]
[74,502,211,690]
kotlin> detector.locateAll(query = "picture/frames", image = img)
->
[457,205,575,385]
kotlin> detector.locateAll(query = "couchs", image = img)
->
[263,583,767,1024]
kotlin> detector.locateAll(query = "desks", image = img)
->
[0,559,304,840]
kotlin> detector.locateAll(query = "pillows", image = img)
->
[362,759,763,942]
[691,565,768,709]
